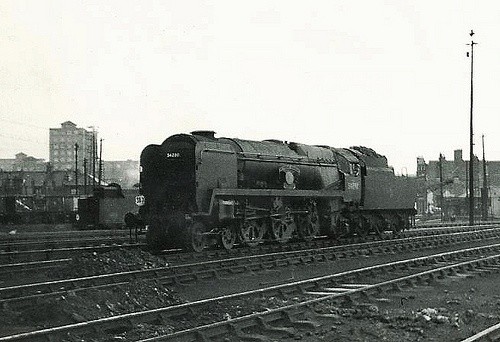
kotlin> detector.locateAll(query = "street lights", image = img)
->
[465,29,479,225]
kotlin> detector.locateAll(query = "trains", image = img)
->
[125,131,420,250]
